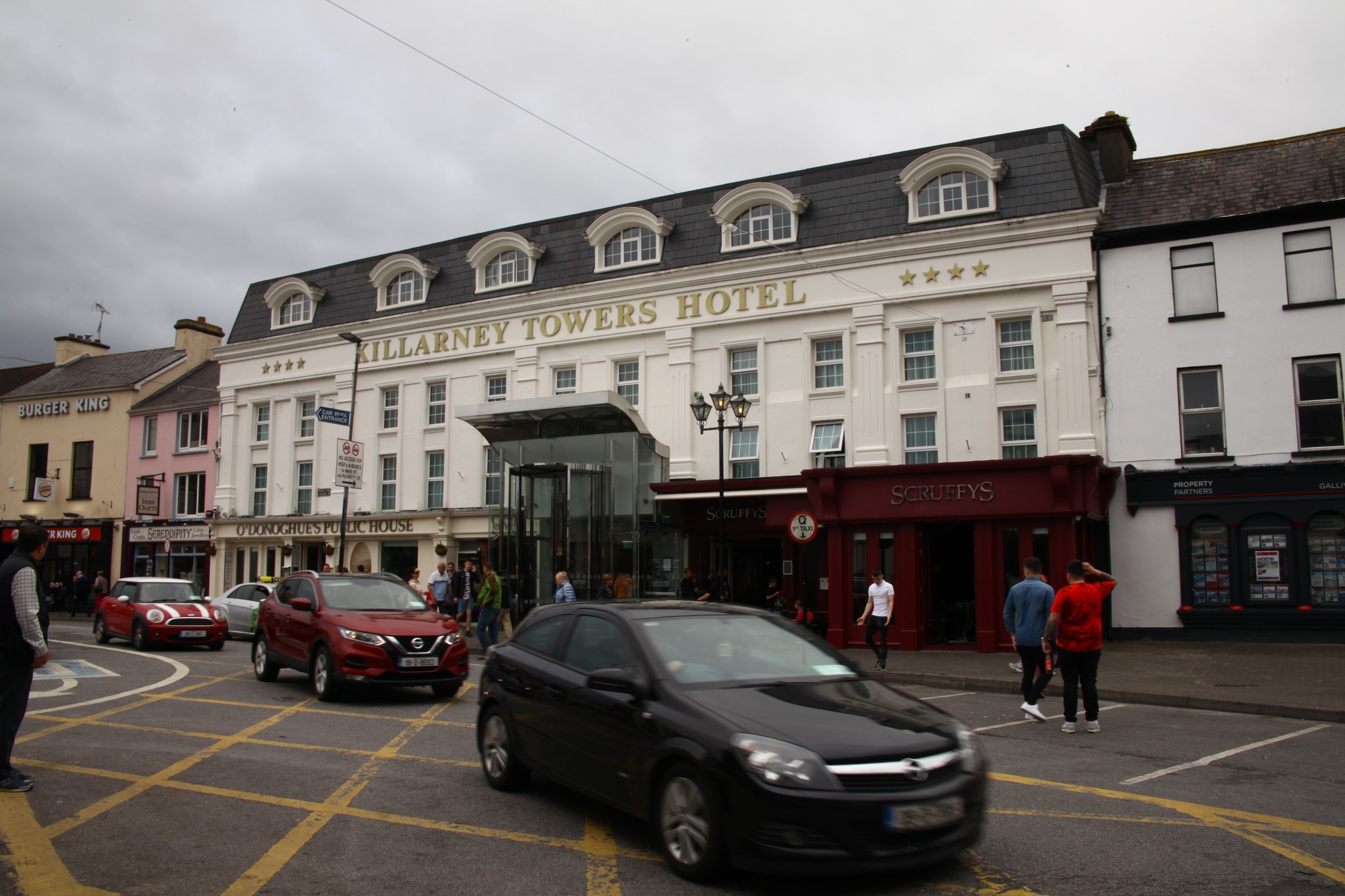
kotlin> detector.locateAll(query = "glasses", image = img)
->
[413,570,420,574]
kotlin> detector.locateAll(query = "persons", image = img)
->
[765,580,783,613]
[857,568,895,671]
[475,561,502,660]
[1009,563,1047,675]
[428,562,452,614]
[596,574,614,600]
[1042,560,1118,734]
[408,566,429,610]
[335,565,350,573]
[357,564,364,574]
[792,599,814,624]
[681,568,698,601]
[0,525,52,792]
[451,559,480,637]
[1003,557,1056,722]
[614,567,634,599]
[70,571,93,618]
[485,570,513,638]
[447,562,457,592]
[553,571,577,603]
[697,566,731,602]
[93,570,110,616]
[323,563,330,573]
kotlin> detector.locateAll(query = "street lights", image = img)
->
[336,331,365,571]
[689,381,753,602]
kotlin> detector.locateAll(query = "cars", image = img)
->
[209,575,286,642]
[477,595,991,896]
[92,576,229,652]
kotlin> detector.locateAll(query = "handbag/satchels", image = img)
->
[425,592,438,612]
[94,582,103,593]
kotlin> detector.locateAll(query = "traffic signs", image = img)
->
[315,404,350,427]
[336,439,365,489]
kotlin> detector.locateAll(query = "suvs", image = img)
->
[251,569,470,703]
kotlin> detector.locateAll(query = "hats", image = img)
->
[1039,574,1047,583]
[496,571,504,576]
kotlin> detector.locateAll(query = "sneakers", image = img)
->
[10,767,35,782]
[0,776,34,792]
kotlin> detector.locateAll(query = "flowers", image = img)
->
[436,540,446,549]
[205,541,216,550]
[278,543,296,550]
[322,541,334,549]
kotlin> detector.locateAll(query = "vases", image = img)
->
[206,549,217,555]
[323,548,334,556]
[435,549,447,556]
[281,548,292,557]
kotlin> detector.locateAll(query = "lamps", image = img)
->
[19,515,36,521]
[63,513,83,517]
[206,510,213,517]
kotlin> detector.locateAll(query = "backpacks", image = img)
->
[572,585,581,601]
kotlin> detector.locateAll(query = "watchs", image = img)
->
[1042,637,1050,642]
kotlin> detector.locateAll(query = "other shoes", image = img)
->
[1009,661,1023,673]
[87,612,92,617]
[1024,712,1035,719]
[478,656,485,660]
[1087,721,1101,733]
[1033,680,1045,698]
[874,659,887,670]
[880,665,887,671]
[1054,659,1061,668]
[1052,672,1055,676]
[1062,723,1076,733]
[1019,677,1027,692]
[466,630,473,637]
[1020,702,1047,721]
[70,615,75,618]
